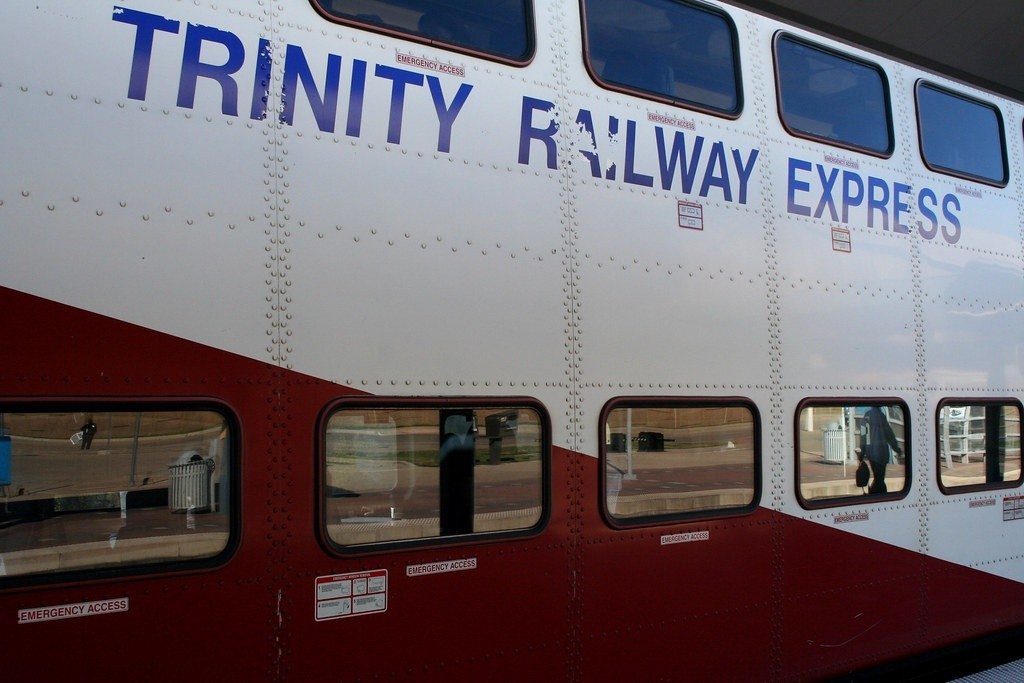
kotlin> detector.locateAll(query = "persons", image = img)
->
[857,406,903,496]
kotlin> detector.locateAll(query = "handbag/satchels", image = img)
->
[855,460,870,487]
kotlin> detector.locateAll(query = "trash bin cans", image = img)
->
[0,435,13,486]
[820,421,845,464]
[165,449,214,515]
